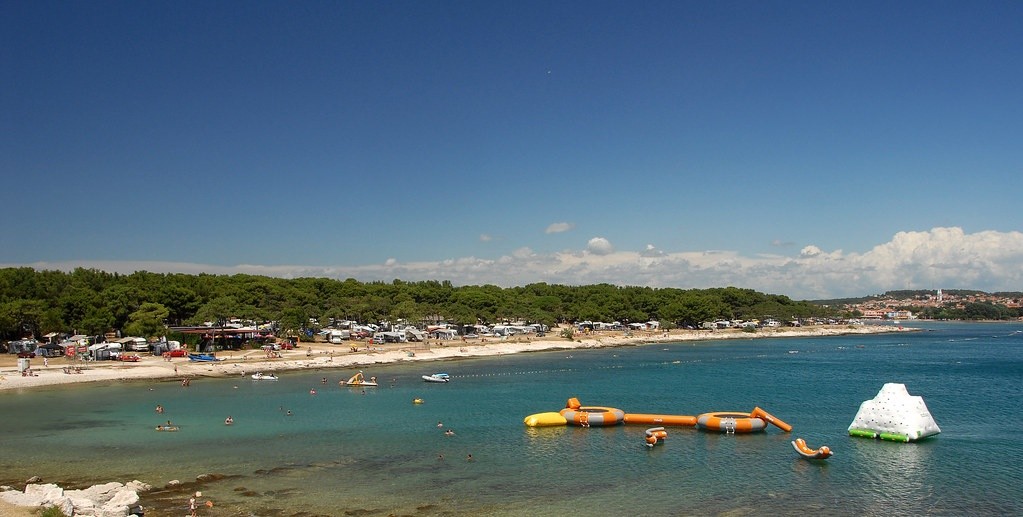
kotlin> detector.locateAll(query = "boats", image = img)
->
[188,354,220,362]
[346,373,378,387]
[422,374,450,382]
[252,374,279,380]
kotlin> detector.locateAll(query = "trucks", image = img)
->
[326,331,342,344]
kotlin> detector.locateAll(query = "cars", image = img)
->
[463,334,478,339]
[163,349,188,358]
[368,337,385,345]
[278,342,292,350]
[260,343,282,351]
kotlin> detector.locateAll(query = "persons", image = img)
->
[182,376,190,386]
[242,370,245,377]
[174,365,178,374]
[189,495,198,515]
[371,377,378,383]
[322,377,328,385]
[22,371,33,376]
[225,416,233,424]
[311,389,317,395]
[158,426,164,431]
[233,385,238,388]
[167,421,171,425]
[44,357,48,366]
[438,422,443,428]
[287,409,291,415]
[439,454,444,458]
[361,391,365,395]
[467,454,473,459]
[339,379,344,386]
[416,396,420,401]
[156,405,163,413]
[445,429,453,435]
